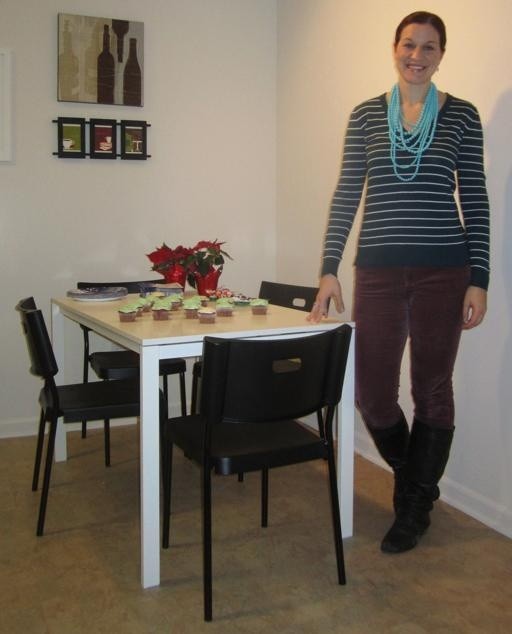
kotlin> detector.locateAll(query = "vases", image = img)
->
[162,265,185,292]
[194,272,221,296]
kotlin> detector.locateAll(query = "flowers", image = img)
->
[148,240,190,274]
[182,239,233,288]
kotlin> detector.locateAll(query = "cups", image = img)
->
[63,139,75,150]
[105,136,111,145]
[117,291,270,324]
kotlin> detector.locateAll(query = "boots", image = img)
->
[381,419,454,553]
[365,408,410,518]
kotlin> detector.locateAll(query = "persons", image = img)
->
[305,10,491,553]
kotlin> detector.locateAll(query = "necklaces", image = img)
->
[387,81,439,182]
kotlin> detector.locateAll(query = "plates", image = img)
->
[68,298,124,302]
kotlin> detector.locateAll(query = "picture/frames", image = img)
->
[57,117,85,158]
[90,118,117,159]
[121,120,147,160]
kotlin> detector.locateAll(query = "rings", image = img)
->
[313,302,319,306]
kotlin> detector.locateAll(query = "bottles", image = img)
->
[57,14,141,106]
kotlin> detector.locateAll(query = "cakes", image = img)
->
[118,291,269,325]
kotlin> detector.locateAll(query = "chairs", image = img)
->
[163,324,347,622]
[193,280,330,482]
[14,297,165,536]
[77,279,187,467]
